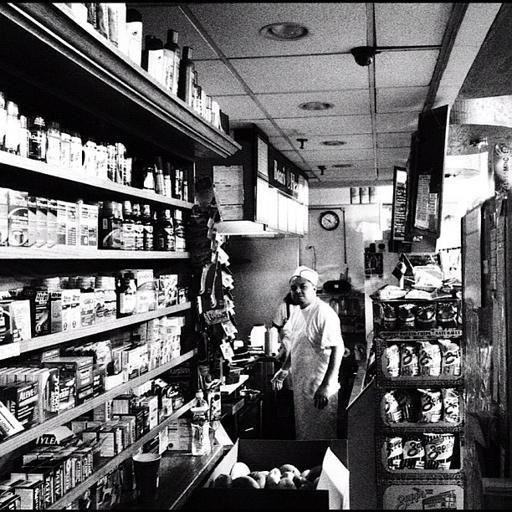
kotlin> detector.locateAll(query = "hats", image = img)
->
[288,265,319,286]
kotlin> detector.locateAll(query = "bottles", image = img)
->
[189,388,212,457]
[95,200,188,253]
[1,93,189,202]
[268,322,279,357]
[250,322,268,354]
[58,2,221,132]
[32,270,155,321]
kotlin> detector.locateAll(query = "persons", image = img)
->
[271,290,299,348]
[270,266,345,441]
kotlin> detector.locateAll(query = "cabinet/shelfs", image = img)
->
[1,18,232,512]
[201,139,310,240]
[370,282,487,510]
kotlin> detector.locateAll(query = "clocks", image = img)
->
[320,211,339,230]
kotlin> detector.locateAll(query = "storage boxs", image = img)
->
[206,438,352,511]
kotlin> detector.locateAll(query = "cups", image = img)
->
[134,454,164,493]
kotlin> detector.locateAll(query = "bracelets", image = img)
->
[273,367,289,383]
